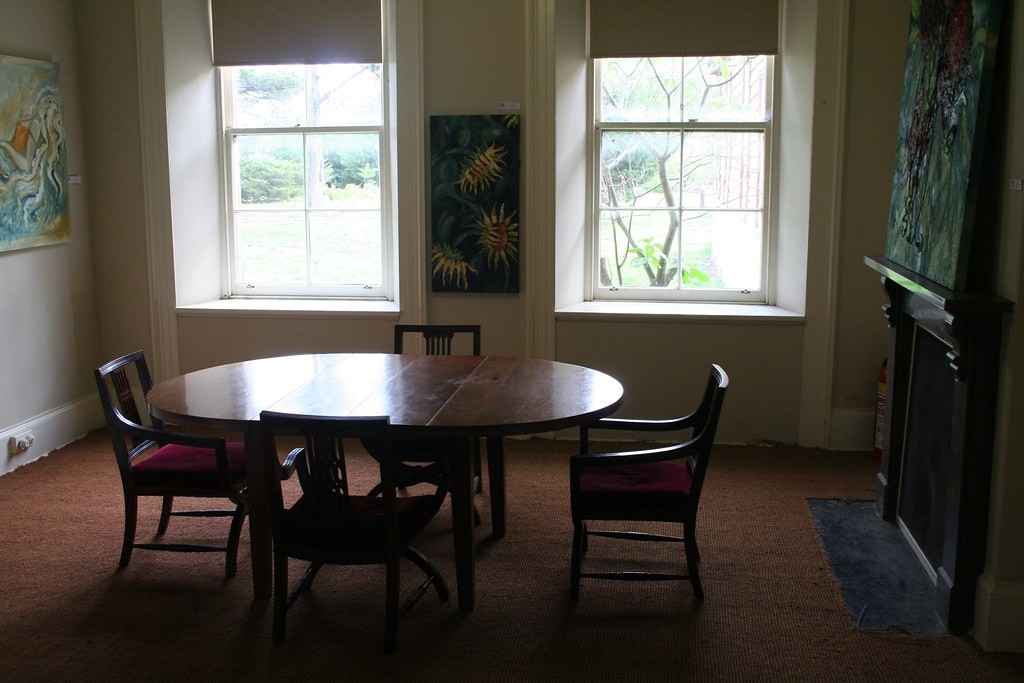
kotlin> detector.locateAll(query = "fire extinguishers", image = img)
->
[872,356,888,464]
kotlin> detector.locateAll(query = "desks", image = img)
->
[144,351,624,612]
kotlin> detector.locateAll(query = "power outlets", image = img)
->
[11,431,34,454]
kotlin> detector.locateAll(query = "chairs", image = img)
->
[258,407,449,654]
[364,322,484,527]
[93,350,247,578]
[570,362,731,606]
